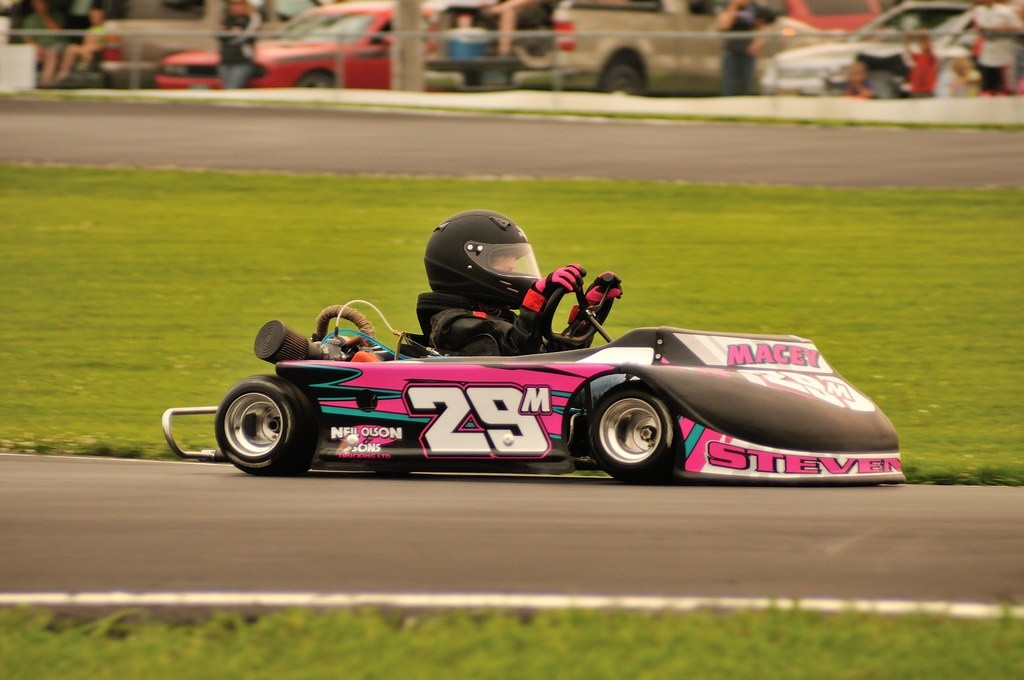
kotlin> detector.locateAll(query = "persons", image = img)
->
[899,14,937,98]
[479,0,561,54]
[21,0,66,88]
[847,62,878,97]
[416,210,623,356]
[714,1,775,96]
[215,0,260,89]
[446,7,480,28]
[57,4,122,80]
[971,0,1024,93]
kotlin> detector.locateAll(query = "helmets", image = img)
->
[424,210,541,309]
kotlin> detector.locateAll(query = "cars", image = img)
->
[84,0,983,98]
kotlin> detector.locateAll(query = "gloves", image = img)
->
[521,264,586,315]
[567,271,625,328]
